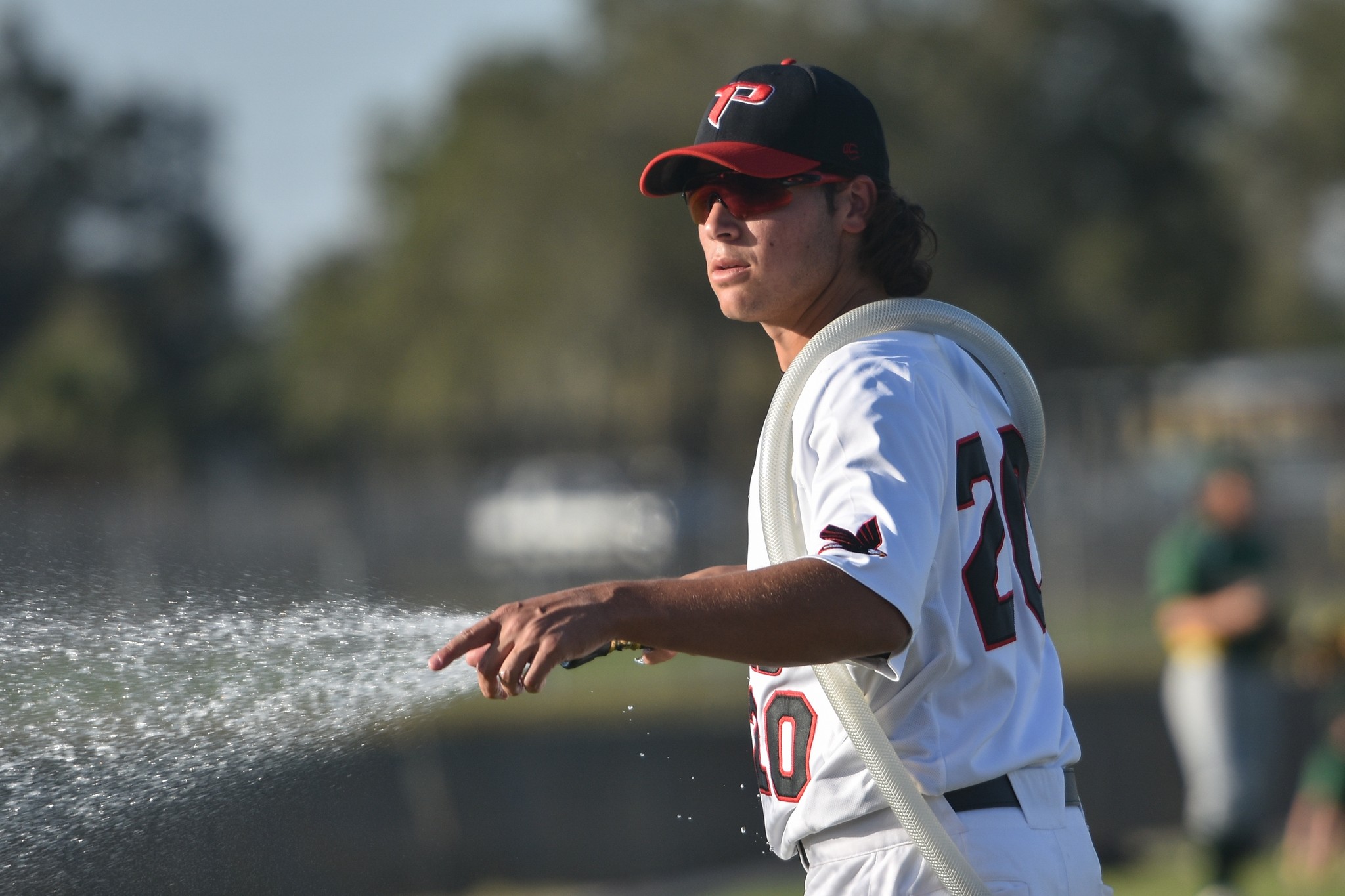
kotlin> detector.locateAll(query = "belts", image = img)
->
[795,766,1080,870]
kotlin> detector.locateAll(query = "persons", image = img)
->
[427,58,1106,896]
[1149,435,1345,896]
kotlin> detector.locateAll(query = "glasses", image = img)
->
[682,161,854,223]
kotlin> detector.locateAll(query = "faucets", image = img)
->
[525,616,654,680]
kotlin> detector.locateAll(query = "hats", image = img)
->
[640,59,891,188]
